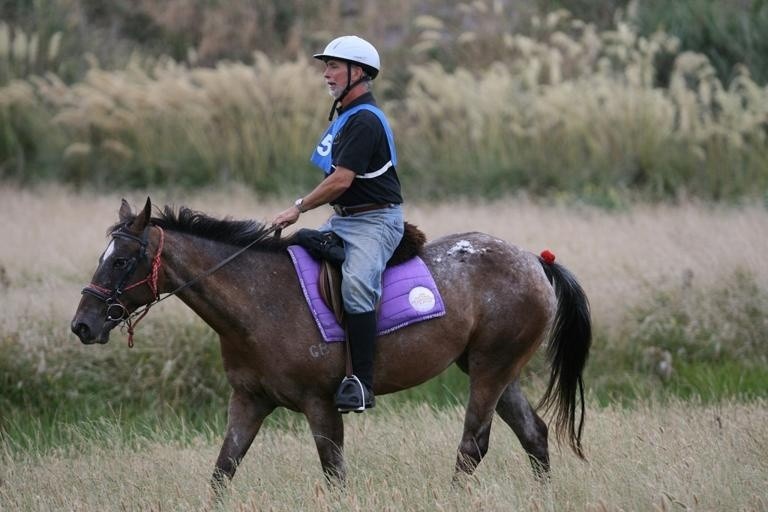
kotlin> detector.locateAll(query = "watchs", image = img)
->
[295,198,308,214]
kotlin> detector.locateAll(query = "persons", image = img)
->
[269,33,406,409]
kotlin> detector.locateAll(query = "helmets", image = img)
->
[314,33,380,75]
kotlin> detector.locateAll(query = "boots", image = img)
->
[333,309,378,406]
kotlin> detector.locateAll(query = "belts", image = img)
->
[332,202,390,217]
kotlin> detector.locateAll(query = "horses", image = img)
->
[69,194,594,512]
